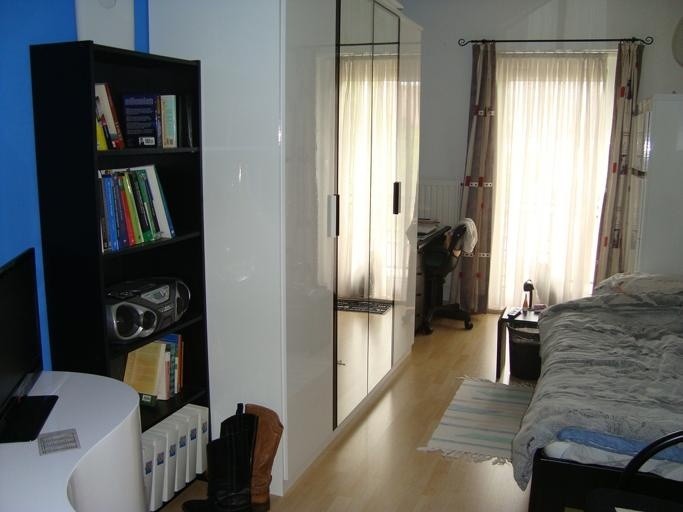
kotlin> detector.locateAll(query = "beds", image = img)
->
[526,294,683,512]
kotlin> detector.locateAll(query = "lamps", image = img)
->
[523,280,536,310]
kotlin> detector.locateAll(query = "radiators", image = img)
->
[419,178,464,304]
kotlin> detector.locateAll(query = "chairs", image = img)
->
[423,218,474,334]
[179,403,285,512]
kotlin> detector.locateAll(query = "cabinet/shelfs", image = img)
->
[27,41,213,512]
[622,93,683,275]
[145,0,423,501]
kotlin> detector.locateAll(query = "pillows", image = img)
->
[594,270,682,306]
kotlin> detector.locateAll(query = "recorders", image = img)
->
[104,276,191,345]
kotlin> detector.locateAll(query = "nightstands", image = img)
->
[495,306,543,380]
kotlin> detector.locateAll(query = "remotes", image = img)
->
[507,307,522,317]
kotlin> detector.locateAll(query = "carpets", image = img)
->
[422,378,539,463]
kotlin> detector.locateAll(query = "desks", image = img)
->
[416,225,451,334]
[0,370,147,512]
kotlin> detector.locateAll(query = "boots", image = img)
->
[182,403,284,512]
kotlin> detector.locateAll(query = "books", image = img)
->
[93,80,194,252]
[417,216,441,235]
[122,332,185,409]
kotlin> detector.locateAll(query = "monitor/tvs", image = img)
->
[0,247,59,442]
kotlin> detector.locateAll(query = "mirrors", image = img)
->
[334,0,402,428]
